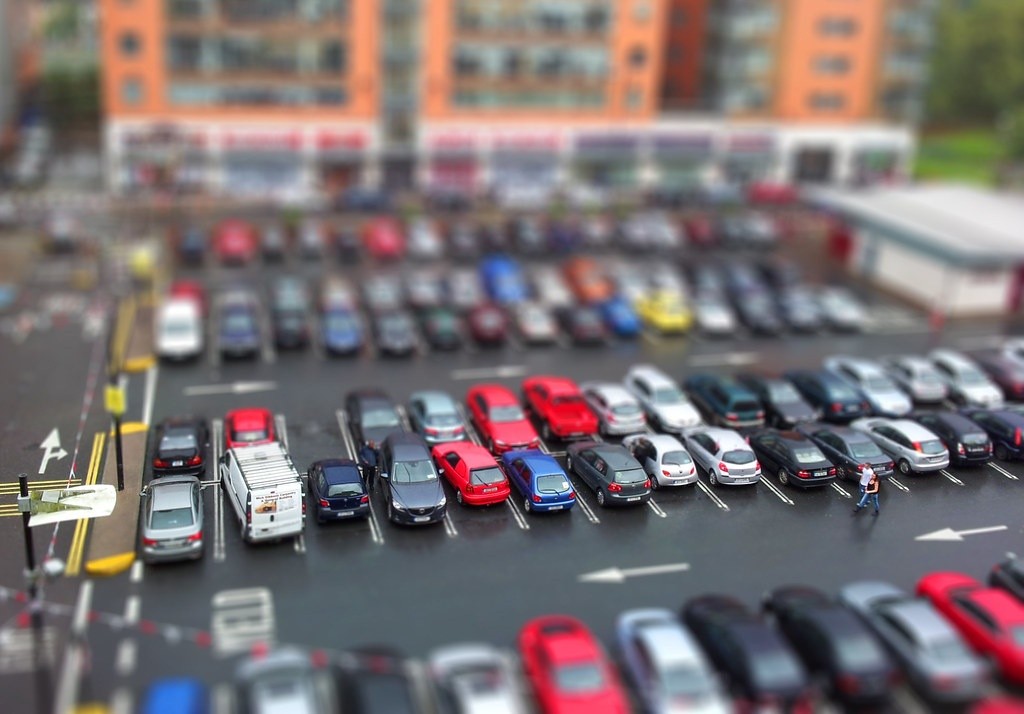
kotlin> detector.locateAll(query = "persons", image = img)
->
[852,462,880,516]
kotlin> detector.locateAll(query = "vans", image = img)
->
[220,440,305,546]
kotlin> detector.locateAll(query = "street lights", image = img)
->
[17,471,117,590]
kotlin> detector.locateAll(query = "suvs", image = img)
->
[374,431,447,528]
[685,371,765,430]
[431,440,512,507]
[626,364,702,434]
[502,447,576,515]
[579,378,646,437]
[565,440,653,508]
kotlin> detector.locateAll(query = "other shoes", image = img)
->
[872,512,879,516]
[852,508,859,513]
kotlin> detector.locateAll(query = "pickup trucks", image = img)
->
[522,373,599,440]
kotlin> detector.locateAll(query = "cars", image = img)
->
[149,182,866,367]
[345,389,405,454]
[138,557,1023,714]
[738,337,1024,491]
[308,457,372,527]
[745,427,837,489]
[140,474,208,566]
[467,385,540,455]
[683,426,762,487]
[406,390,469,445]
[151,414,209,478]
[622,433,699,490]
[224,408,275,452]
